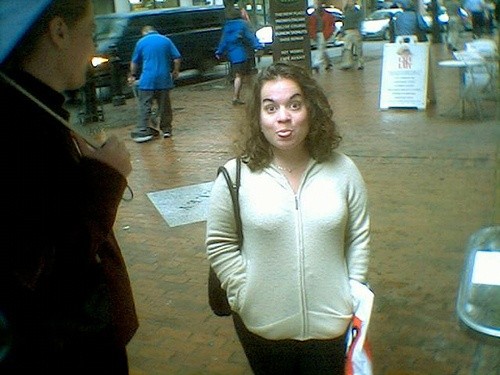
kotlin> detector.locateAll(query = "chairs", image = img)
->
[452,39,499,120]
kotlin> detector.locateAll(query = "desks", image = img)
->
[438,60,484,119]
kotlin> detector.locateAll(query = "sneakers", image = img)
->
[130,129,172,142]
[232,97,245,105]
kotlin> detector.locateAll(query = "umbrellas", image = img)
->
[0,0,133,201]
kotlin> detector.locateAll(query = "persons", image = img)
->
[127,25,180,143]
[464,0,494,39]
[339,0,363,69]
[307,2,334,71]
[215,9,264,104]
[206,62,370,375]
[0,0,139,375]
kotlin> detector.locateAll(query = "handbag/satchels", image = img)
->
[344,275,375,375]
[207,156,241,317]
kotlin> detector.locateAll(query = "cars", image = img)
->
[362,6,473,41]
[257,6,345,53]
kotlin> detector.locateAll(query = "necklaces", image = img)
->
[275,163,308,172]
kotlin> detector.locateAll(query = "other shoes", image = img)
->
[324,64,333,71]
[312,66,320,72]
[339,67,352,70]
[357,66,364,70]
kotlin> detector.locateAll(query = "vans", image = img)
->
[64,6,228,98]
